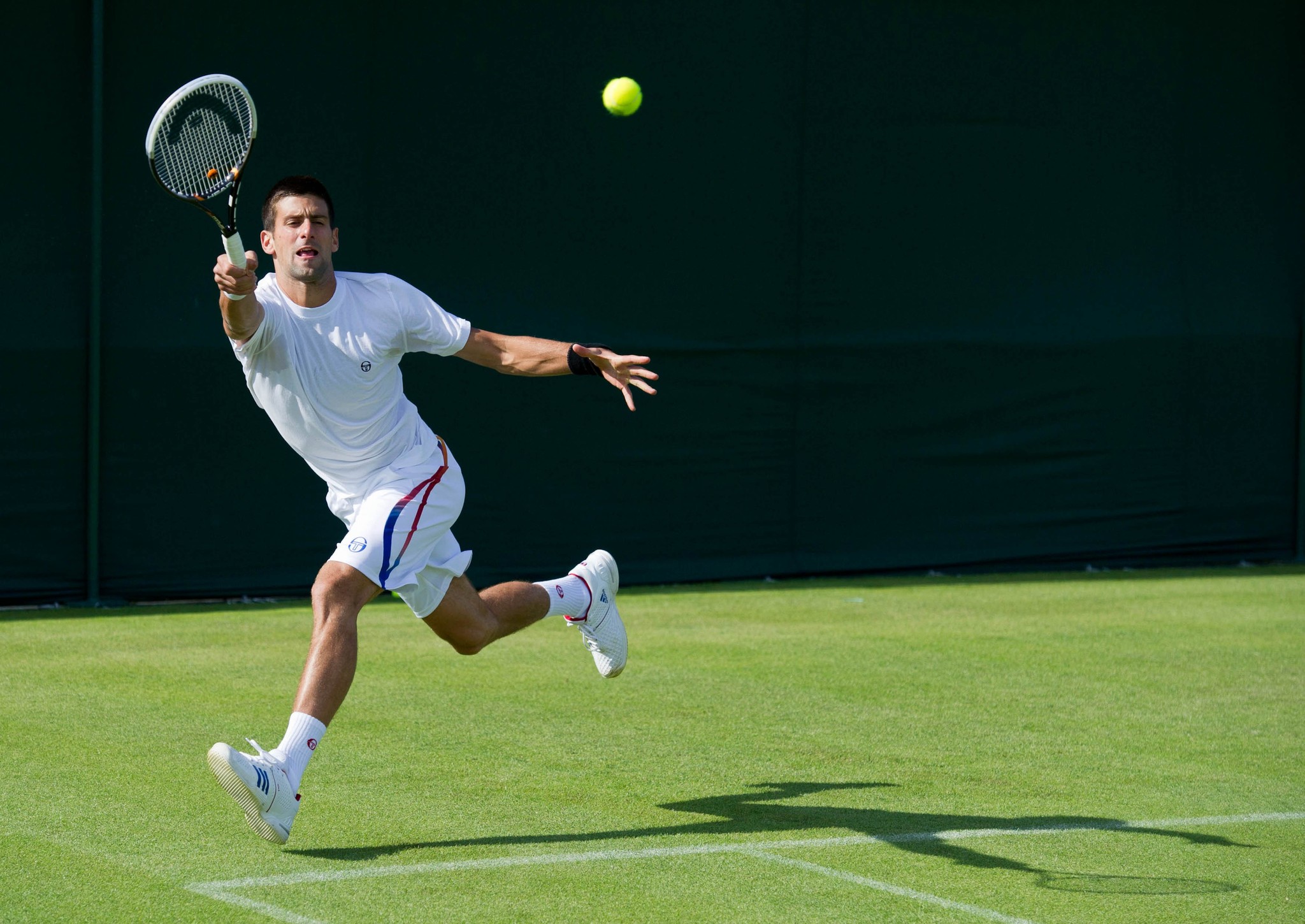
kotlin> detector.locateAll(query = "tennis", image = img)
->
[602,76,641,116]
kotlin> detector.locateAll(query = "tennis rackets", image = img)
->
[145,72,257,268]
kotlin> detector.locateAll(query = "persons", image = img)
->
[205,174,658,844]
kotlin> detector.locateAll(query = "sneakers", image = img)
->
[563,550,626,679]
[207,742,302,845]
